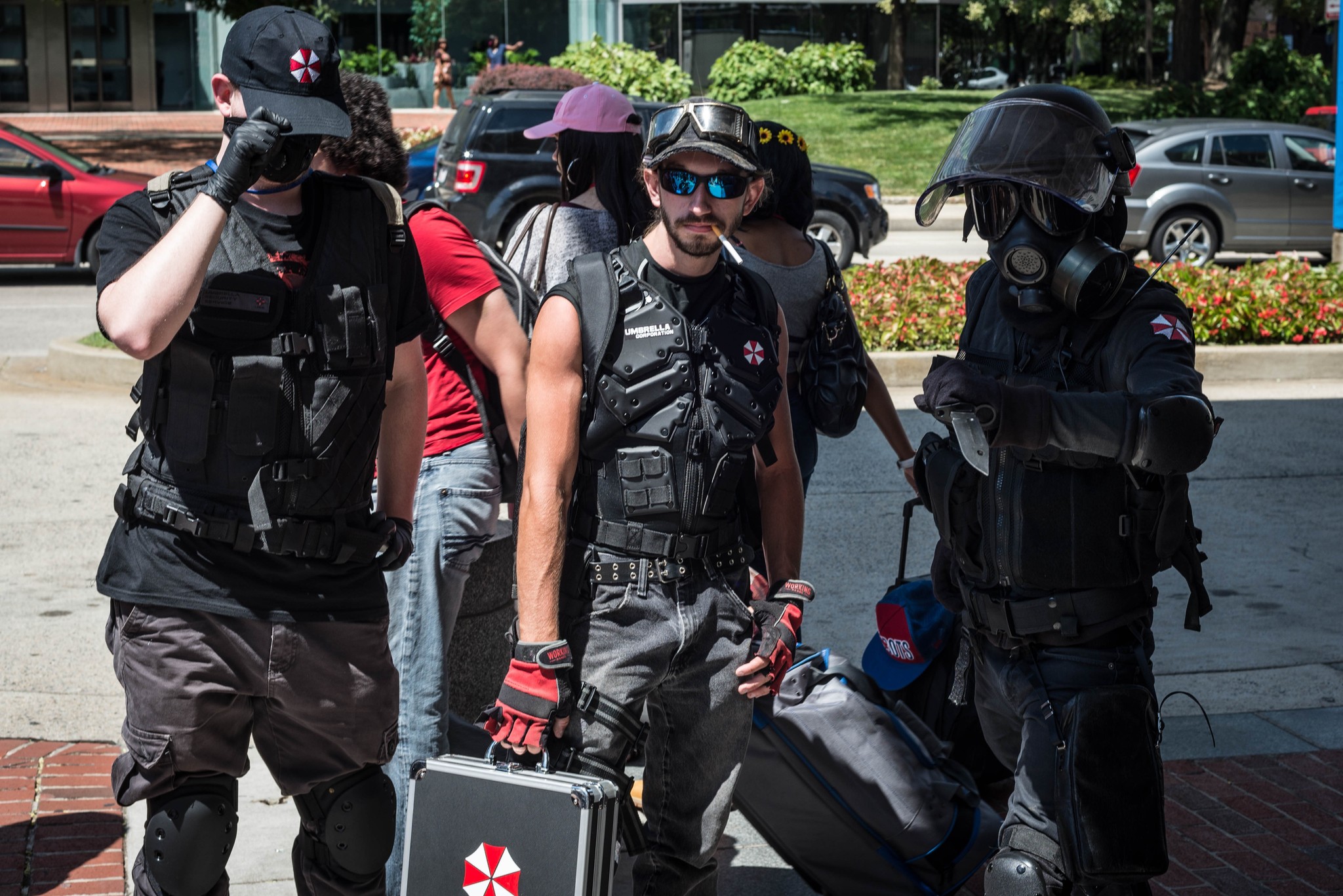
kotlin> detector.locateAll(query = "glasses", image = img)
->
[641,103,755,156]
[949,168,1096,238]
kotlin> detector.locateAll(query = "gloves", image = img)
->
[192,105,292,215]
[369,511,415,572]
[916,347,1050,455]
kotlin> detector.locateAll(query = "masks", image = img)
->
[221,116,324,185]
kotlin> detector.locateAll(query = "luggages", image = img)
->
[736,636,1002,892]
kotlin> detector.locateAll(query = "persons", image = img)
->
[486,34,524,74]
[474,97,818,895]
[308,71,531,896]
[913,79,1223,894]
[500,81,658,343]
[724,120,923,519]
[94,6,437,895]
[431,37,459,110]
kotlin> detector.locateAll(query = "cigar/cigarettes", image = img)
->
[711,224,743,265]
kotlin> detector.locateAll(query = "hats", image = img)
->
[223,3,355,139]
[523,81,641,139]
[645,95,762,172]
[862,570,950,697]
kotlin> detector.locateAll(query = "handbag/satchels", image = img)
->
[805,236,868,439]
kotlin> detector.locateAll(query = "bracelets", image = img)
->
[896,448,919,470]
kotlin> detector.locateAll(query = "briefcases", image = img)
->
[395,731,621,895]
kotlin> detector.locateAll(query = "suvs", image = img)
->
[431,85,889,267]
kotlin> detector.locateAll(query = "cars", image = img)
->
[1107,117,1337,265]
[2,120,158,278]
[955,67,1012,90]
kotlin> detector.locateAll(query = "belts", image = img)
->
[586,543,750,587]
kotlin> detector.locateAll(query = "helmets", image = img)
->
[916,86,1135,173]
